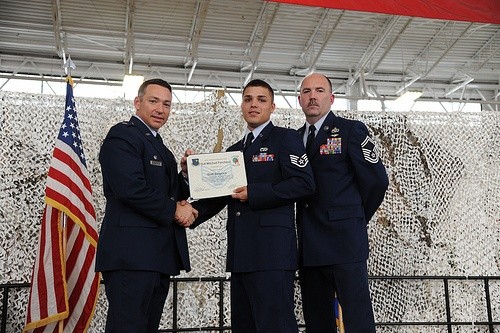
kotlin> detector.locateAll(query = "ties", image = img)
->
[243,132,254,155]
[306,125,316,152]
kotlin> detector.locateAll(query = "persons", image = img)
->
[173,78,316,333]
[94,78,197,333]
[290,72,389,333]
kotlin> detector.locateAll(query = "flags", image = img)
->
[22,77,100,333]
